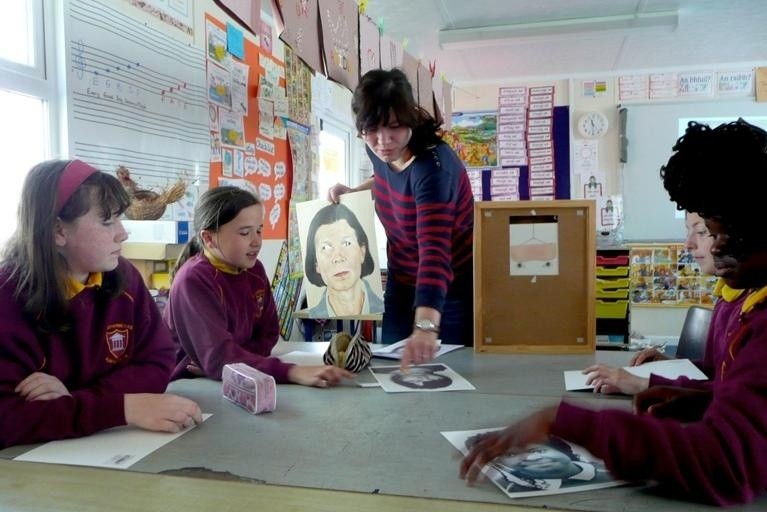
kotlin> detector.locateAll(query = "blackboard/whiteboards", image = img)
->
[617,95,767,243]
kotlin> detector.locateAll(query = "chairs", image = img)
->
[674,306,715,358]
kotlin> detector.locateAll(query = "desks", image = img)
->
[0,343,764,512]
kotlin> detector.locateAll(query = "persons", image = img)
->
[579,212,752,399]
[458,121,766,507]
[368,360,454,389]
[629,245,717,309]
[325,68,476,377]
[0,157,205,447]
[460,426,621,491]
[304,199,385,320]
[162,185,356,389]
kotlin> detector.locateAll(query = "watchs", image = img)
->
[411,318,439,333]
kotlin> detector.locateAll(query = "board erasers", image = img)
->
[618,108,628,163]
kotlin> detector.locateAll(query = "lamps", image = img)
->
[438,8,679,52]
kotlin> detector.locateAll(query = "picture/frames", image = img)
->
[472,199,597,356]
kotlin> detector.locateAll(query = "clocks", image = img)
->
[577,110,608,140]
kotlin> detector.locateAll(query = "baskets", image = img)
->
[124,189,166,220]
